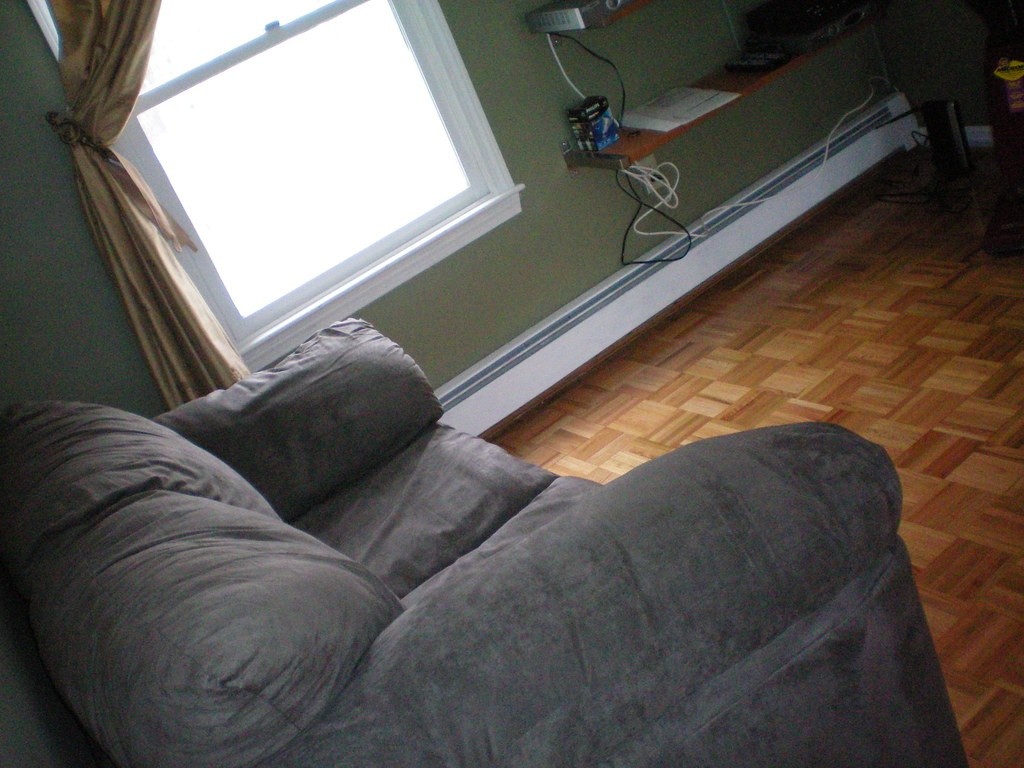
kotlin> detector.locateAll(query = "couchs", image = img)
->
[0,316,974,768]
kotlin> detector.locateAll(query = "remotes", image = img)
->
[725,47,792,74]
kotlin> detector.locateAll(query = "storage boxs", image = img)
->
[567,96,621,151]
